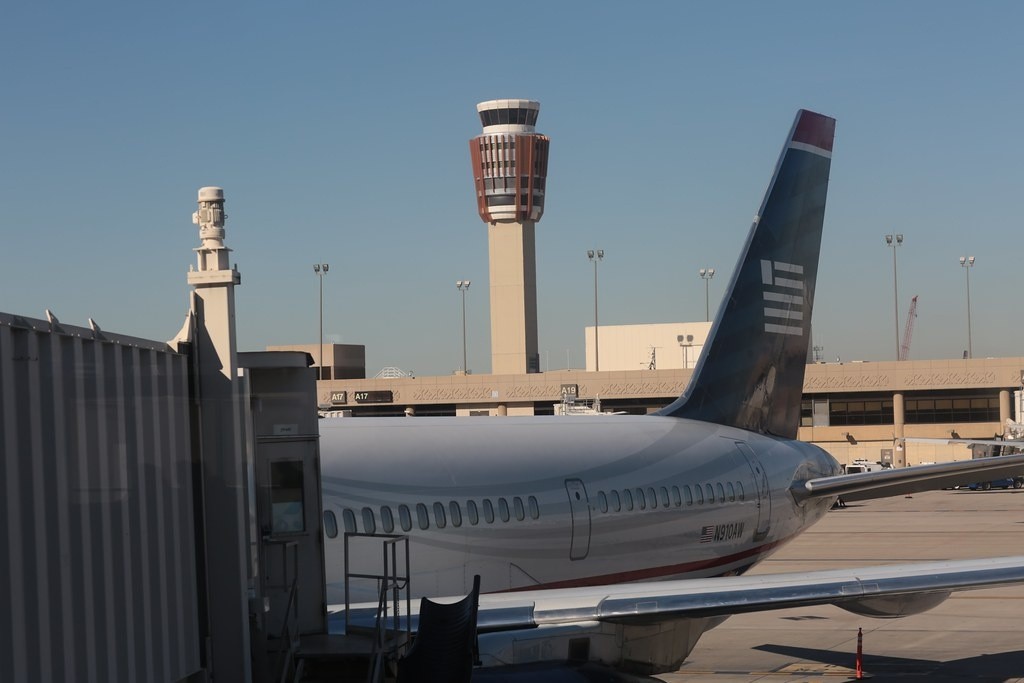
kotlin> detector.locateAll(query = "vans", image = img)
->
[845,459,886,475]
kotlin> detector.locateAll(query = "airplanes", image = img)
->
[321,109,1023,682]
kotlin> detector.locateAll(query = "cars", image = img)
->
[940,475,1024,491]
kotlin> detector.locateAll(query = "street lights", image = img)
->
[885,234,903,360]
[587,249,605,371]
[314,262,329,379]
[455,280,472,377]
[700,268,715,322]
[959,255,975,357]
